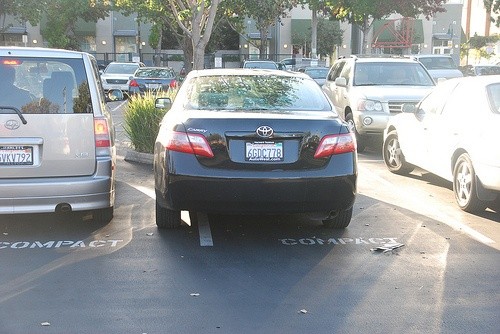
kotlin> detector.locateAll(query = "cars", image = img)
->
[154,69,358,231]
[126,66,179,103]
[239,58,279,70]
[98,60,145,102]
[278,54,331,87]
[381,74,500,213]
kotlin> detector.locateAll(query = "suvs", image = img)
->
[404,53,464,86]
[322,53,439,152]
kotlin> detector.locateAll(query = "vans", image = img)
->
[0,44,124,228]
